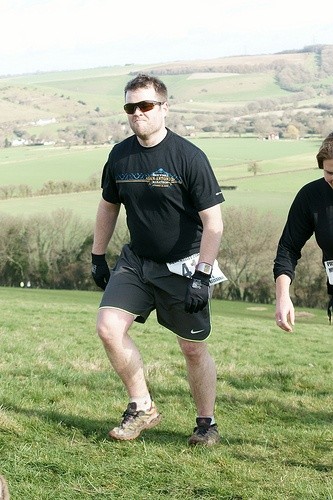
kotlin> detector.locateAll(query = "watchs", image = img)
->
[195,261,213,275]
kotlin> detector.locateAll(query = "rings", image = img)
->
[278,318,282,323]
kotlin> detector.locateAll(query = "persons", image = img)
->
[89,74,225,447]
[273,132,333,335]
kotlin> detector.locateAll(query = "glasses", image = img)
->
[124,101,161,114]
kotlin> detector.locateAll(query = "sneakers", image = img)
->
[109,400,161,440]
[188,417,221,446]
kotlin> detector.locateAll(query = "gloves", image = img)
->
[91,252,110,289]
[186,262,212,313]
